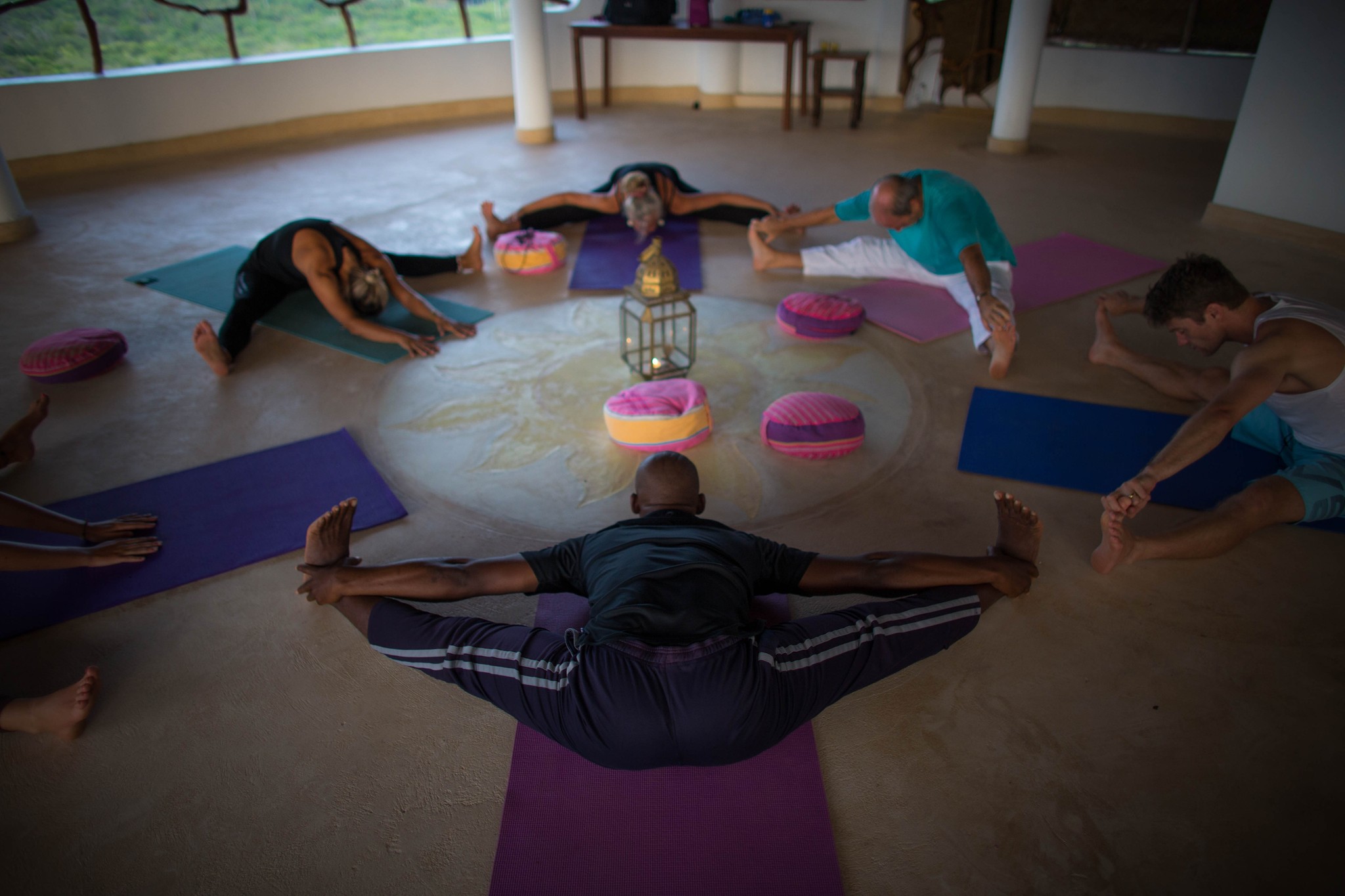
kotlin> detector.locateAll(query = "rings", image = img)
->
[1128,493,1135,498]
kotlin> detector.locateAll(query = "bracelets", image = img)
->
[83,521,88,538]
[976,293,987,306]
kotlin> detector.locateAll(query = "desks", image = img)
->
[567,20,812,130]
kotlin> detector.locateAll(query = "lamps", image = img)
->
[619,243,697,383]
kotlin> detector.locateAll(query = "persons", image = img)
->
[483,162,806,235]
[193,218,482,376]
[296,449,1038,771]
[0,391,163,740]
[1088,256,1345,572]
[748,168,1020,379]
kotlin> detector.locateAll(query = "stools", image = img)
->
[807,49,871,129]
